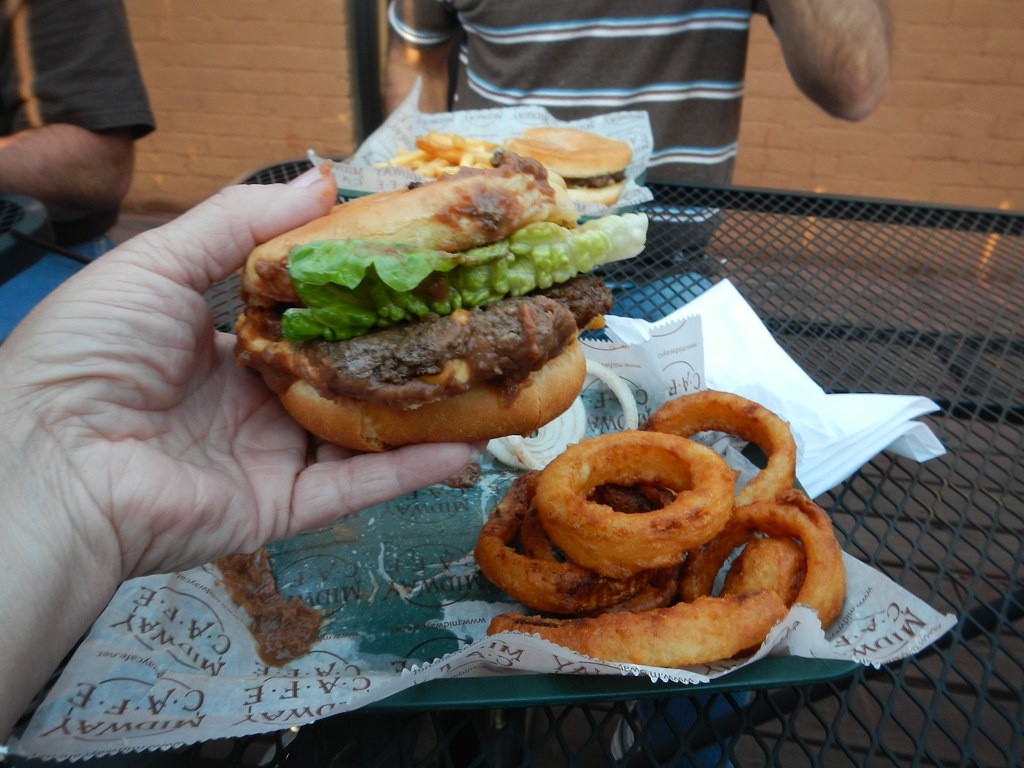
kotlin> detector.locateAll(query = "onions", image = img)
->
[486,362,640,471]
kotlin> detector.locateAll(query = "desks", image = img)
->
[0,155,1024,768]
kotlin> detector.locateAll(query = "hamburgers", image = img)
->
[227,176,648,453]
[505,126,633,204]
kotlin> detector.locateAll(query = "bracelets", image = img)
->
[388,1,454,44]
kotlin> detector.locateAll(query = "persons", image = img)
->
[382,0,892,322]
[0,0,157,347]
[0,167,471,749]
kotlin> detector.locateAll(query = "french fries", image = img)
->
[373,133,503,178]
